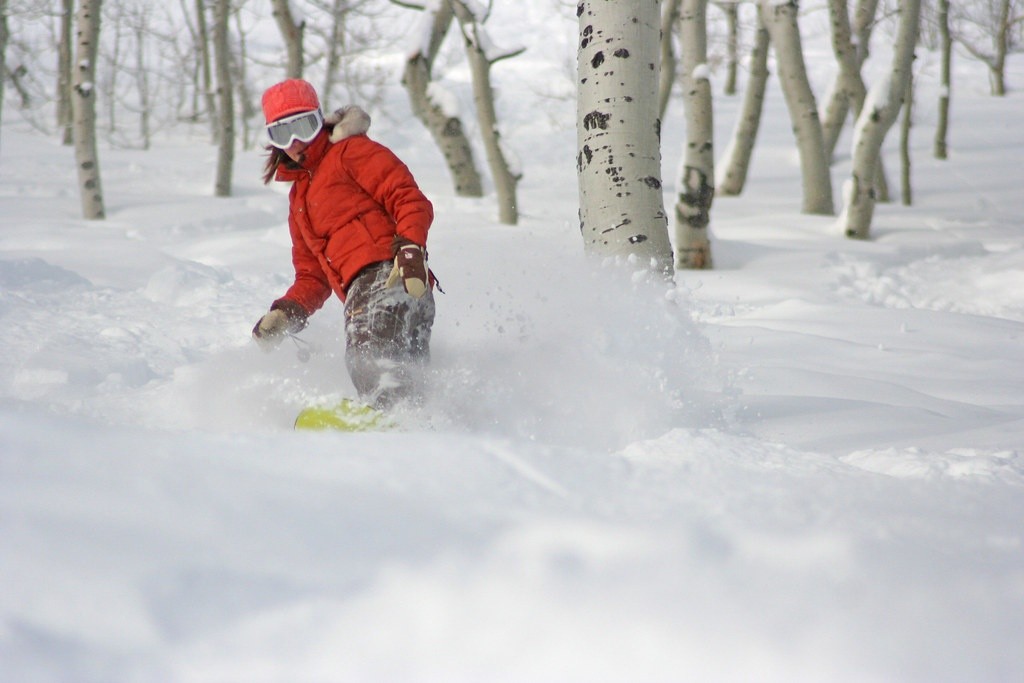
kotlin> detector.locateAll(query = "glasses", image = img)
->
[264,107,324,149]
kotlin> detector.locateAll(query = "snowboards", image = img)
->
[292,399,400,432]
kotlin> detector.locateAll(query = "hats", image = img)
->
[262,78,319,125]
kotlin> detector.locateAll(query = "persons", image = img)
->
[253,76,439,429]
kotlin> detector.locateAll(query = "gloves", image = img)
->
[252,298,307,348]
[385,238,428,296]
